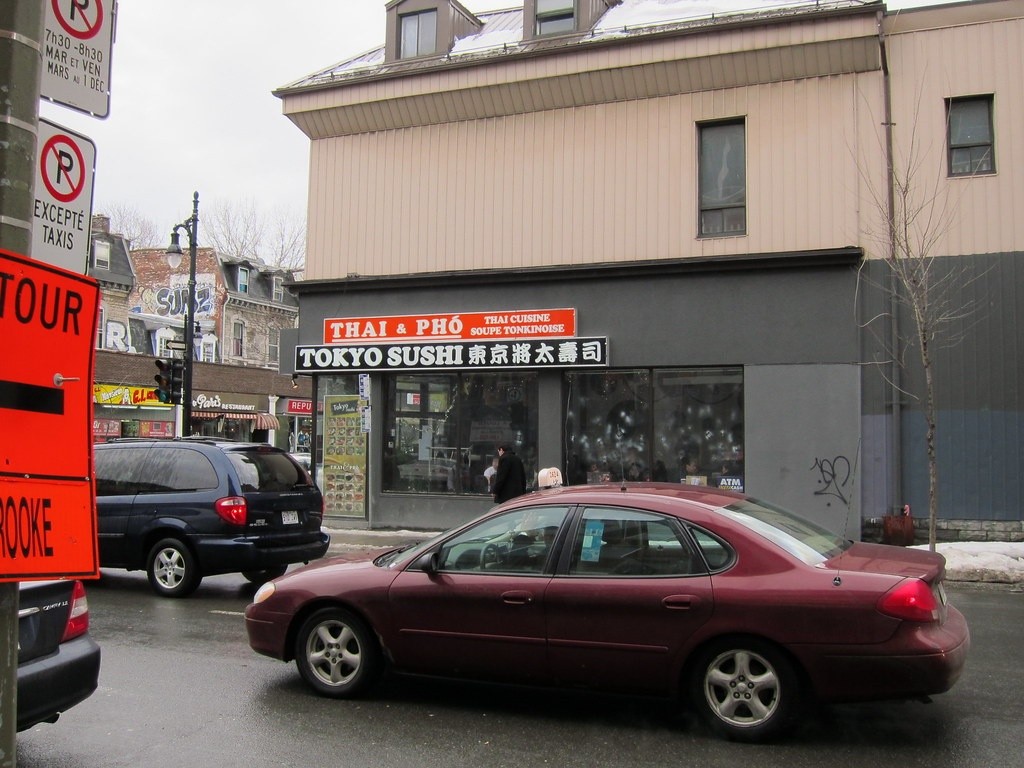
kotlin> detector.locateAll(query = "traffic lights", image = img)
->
[153,358,171,403]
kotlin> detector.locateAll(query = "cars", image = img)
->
[13,578,103,737]
[240,480,972,745]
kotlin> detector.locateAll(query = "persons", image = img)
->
[493,446,526,505]
[673,457,701,482]
[289,433,294,446]
[298,431,304,444]
[484,456,499,492]
[304,432,309,443]
[436,443,452,459]
[627,460,667,482]
[720,460,734,475]
[508,511,560,570]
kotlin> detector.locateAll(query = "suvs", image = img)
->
[91,434,333,601]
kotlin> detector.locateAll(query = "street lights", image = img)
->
[164,189,201,437]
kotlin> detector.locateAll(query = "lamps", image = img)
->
[291,373,298,389]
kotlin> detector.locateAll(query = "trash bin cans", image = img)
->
[883,515,914,546]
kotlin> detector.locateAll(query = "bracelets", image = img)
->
[518,532,527,536]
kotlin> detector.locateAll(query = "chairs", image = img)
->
[474,475,488,494]
[599,518,639,575]
[412,479,431,491]
[399,477,411,492]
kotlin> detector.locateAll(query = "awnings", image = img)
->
[254,412,279,430]
